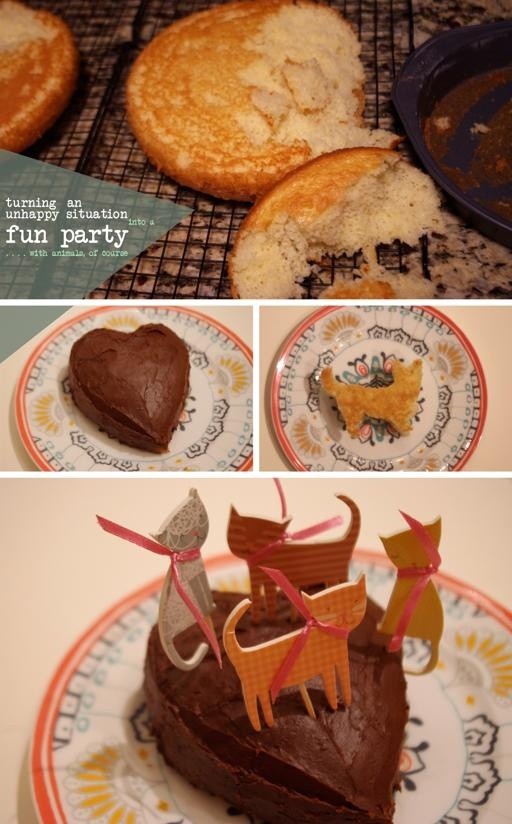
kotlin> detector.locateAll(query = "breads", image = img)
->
[321,359,422,437]
[230,147,444,298]
[0,0,79,153]
[125,1,401,203]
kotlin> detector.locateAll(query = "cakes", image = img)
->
[145,489,445,822]
[69,324,189,452]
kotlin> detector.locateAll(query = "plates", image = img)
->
[267,306,490,472]
[391,21,511,248]
[30,544,511,823]
[12,306,252,472]
